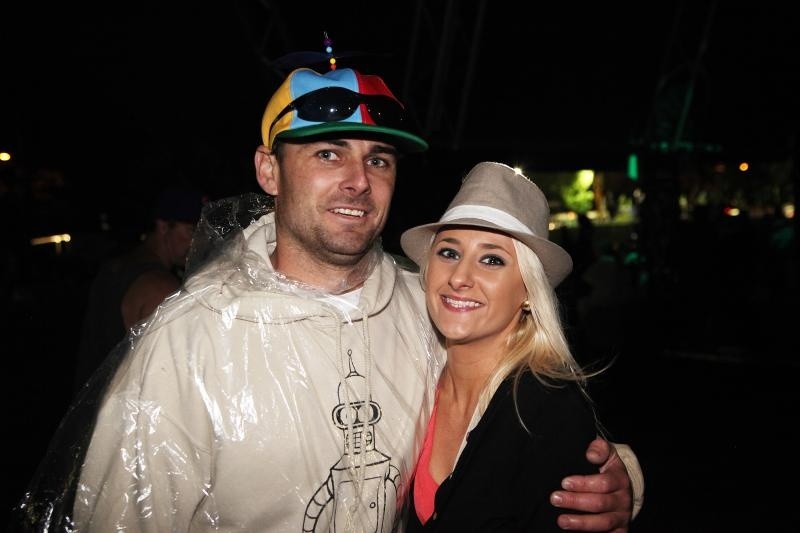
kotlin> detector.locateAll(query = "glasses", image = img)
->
[268,87,406,142]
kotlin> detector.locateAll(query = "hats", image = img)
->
[400,161,572,289]
[150,187,222,225]
[261,68,429,150]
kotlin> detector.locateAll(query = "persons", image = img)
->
[62,69,644,533]
[398,163,610,533]
